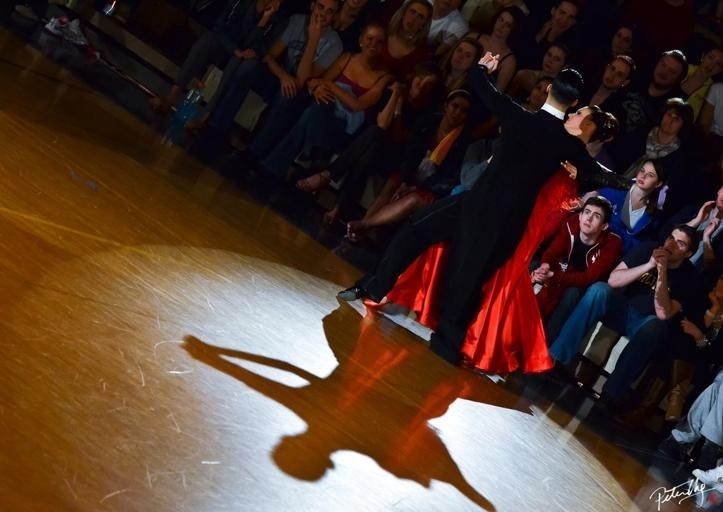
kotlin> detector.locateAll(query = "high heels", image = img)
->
[296,170,341,231]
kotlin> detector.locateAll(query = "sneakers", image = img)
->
[592,391,612,412]
[44,16,88,46]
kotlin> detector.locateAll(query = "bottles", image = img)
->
[174,81,205,122]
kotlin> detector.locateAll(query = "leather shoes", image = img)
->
[338,286,367,301]
[429,340,465,367]
